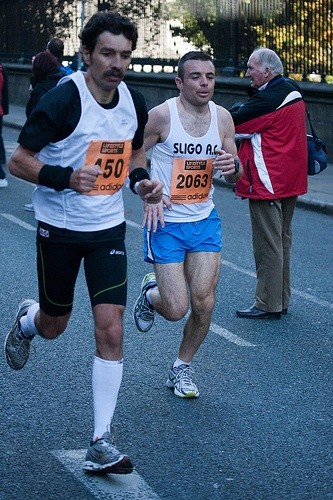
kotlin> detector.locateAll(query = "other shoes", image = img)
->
[0,178,9,188]
[24,204,35,212]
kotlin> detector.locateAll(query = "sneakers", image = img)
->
[165,364,200,398]
[133,273,155,332]
[82,431,133,476]
[3,299,37,371]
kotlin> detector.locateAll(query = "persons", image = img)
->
[134,52,246,398]
[0,38,76,211]
[227,49,309,320]
[4,13,165,473]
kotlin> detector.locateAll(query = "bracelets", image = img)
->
[129,168,151,195]
[233,159,240,174]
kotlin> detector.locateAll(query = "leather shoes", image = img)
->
[281,308,289,314]
[235,303,282,320]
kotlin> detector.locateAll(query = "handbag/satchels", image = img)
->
[305,135,329,176]
